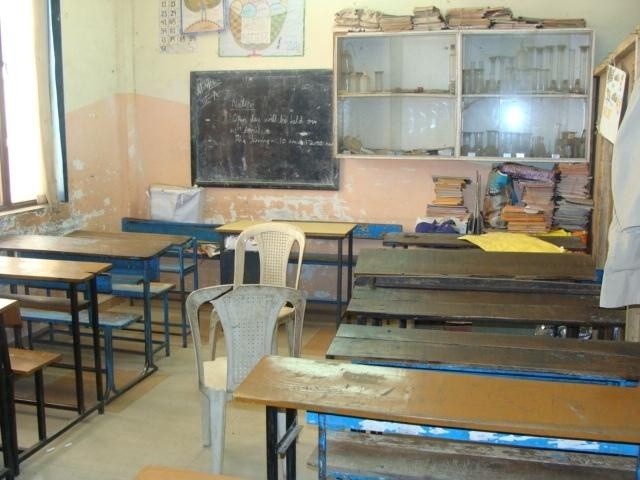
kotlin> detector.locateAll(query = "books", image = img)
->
[330,5,587,31]
[424,173,474,222]
[188,237,221,260]
[553,161,595,233]
[483,164,555,233]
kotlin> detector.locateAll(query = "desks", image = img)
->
[215,219,357,330]
[1,232,173,374]
[0,253,114,424]
[233,354,638,480]
[64,230,196,350]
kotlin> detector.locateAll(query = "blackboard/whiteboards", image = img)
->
[188,70,341,191]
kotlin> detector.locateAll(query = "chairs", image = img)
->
[185,284,306,474]
[204,223,304,362]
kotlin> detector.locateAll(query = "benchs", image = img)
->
[6,342,65,454]
[103,277,171,360]
[328,247,640,386]
[13,307,143,406]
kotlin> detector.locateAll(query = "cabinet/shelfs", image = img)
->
[331,27,593,164]
